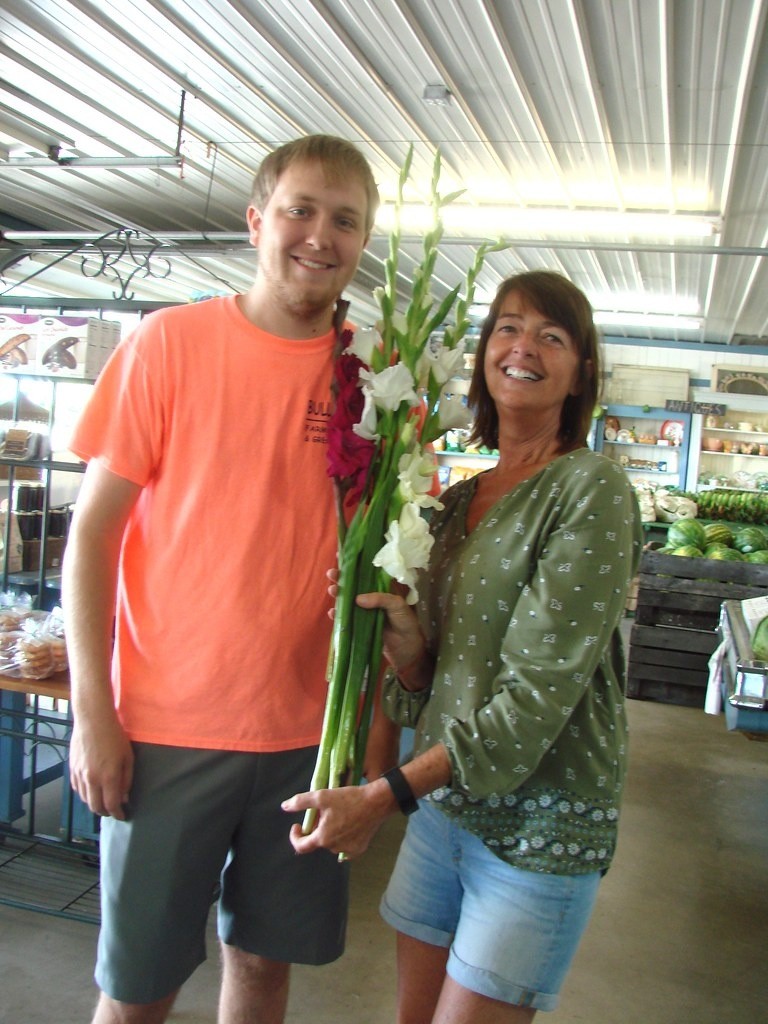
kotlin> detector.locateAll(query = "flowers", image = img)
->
[301,141,511,837]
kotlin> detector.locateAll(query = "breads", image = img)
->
[0,333,80,369]
[0,608,70,680]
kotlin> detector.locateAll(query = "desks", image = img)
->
[0,671,101,925]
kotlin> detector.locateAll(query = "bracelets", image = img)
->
[380,765,420,817]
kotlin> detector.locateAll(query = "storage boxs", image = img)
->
[626,540,768,709]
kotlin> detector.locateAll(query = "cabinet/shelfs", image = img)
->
[423,390,768,495]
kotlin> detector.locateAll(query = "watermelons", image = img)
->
[649,516,768,588]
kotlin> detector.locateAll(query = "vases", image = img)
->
[740,442,752,454]
[723,440,732,452]
[759,444,767,455]
[750,442,760,455]
[703,438,722,452]
[731,441,741,453]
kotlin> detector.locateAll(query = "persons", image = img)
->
[61,134,442,1024]
[283,272,644,1024]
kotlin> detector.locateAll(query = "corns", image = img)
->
[695,491,768,523]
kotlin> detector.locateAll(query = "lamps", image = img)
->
[0,90,186,166]
